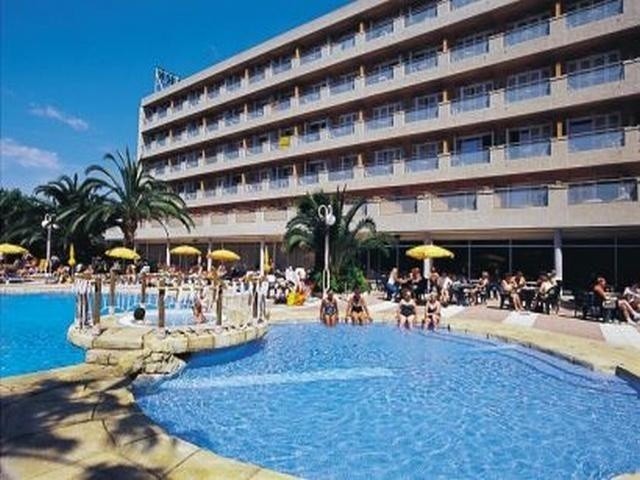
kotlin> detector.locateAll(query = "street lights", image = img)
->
[318,202,338,300]
[40,211,61,273]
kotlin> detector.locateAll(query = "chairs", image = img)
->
[0,261,137,286]
[138,263,231,292]
[382,270,640,324]
[234,265,309,297]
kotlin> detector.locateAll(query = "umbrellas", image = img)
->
[405,244,455,293]
[0,243,29,263]
[169,245,202,274]
[206,248,241,271]
[67,242,77,281]
[264,244,271,275]
[104,246,141,274]
